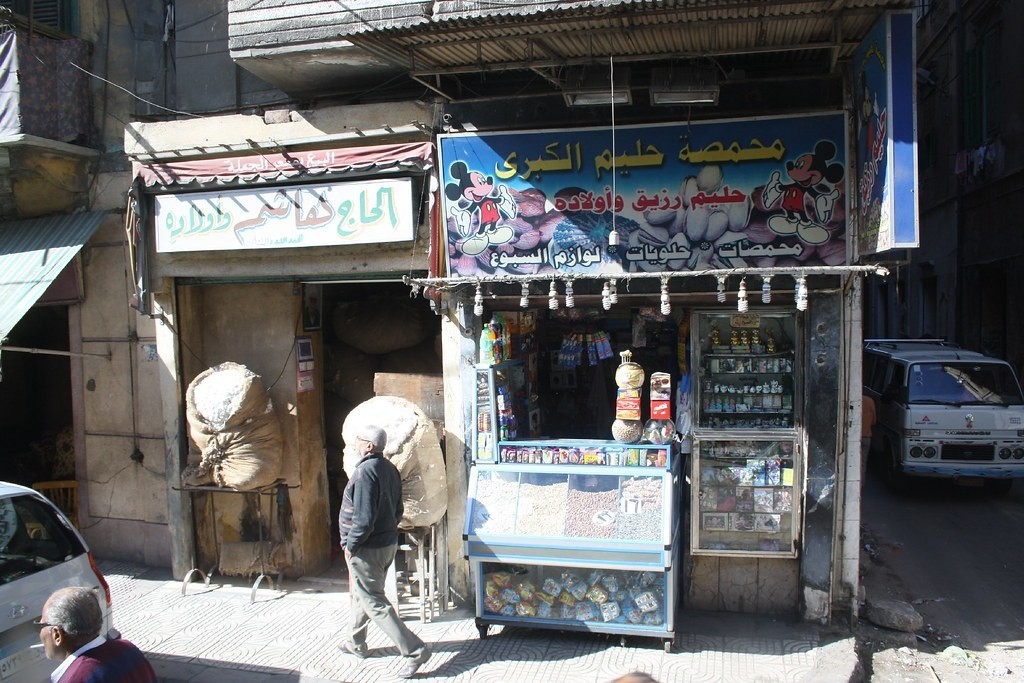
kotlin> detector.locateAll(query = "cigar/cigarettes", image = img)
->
[30,644,44,648]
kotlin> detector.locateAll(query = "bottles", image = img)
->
[478,311,512,365]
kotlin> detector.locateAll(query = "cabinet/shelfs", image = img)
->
[378,520,450,624]
[691,309,804,559]
[462,453,684,653]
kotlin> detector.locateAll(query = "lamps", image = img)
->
[562,86,634,106]
[649,85,721,107]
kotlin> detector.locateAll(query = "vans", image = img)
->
[863,338,1024,497]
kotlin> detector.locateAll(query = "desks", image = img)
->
[173,478,287,603]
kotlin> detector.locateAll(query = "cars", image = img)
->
[0,480,122,683]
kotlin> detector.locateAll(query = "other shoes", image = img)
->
[338,639,369,657]
[399,648,433,679]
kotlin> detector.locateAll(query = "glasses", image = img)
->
[355,435,372,444]
[34,617,54,631]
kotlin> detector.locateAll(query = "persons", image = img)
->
[337,426,432,679]
[860,389,877,489]
[34,587,156,683]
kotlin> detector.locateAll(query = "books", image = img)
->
[700,459,792,533]
